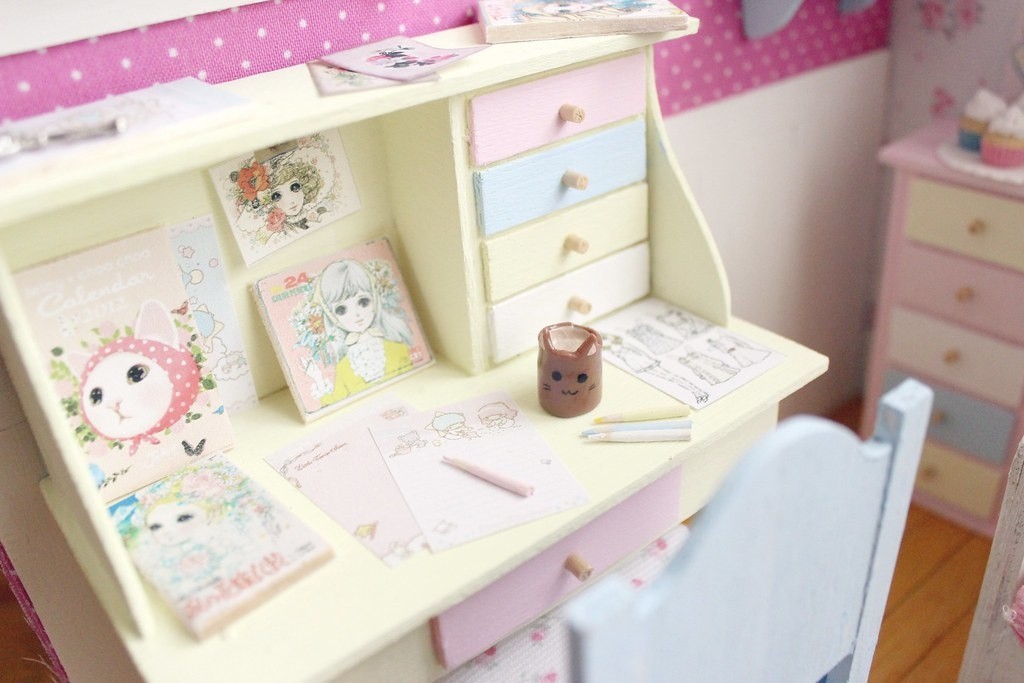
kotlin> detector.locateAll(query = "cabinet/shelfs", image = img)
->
[860,120,1022,538]
[381,44,654,373]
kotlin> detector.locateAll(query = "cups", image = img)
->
[537,323,601,417]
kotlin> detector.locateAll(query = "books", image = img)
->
[104,449,337,647]
[478,0,690,45]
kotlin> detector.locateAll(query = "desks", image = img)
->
[0,18,830,683]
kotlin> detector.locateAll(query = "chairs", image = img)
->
[441,377,934,683]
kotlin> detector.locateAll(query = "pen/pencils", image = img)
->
[583,428,691,442]
[443,454,534,498]
[581,420,692,437]
[595,406,689,424]
